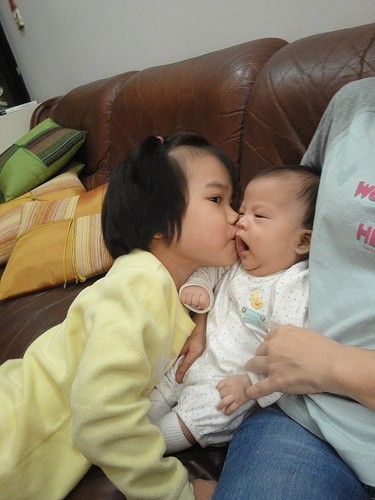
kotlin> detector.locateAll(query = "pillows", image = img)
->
[0,117,115,300]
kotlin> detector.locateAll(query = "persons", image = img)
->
[143,165,320,456]
[174,74,375,500]
[0,132,239,500]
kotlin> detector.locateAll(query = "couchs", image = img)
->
[0,23,375,500]
